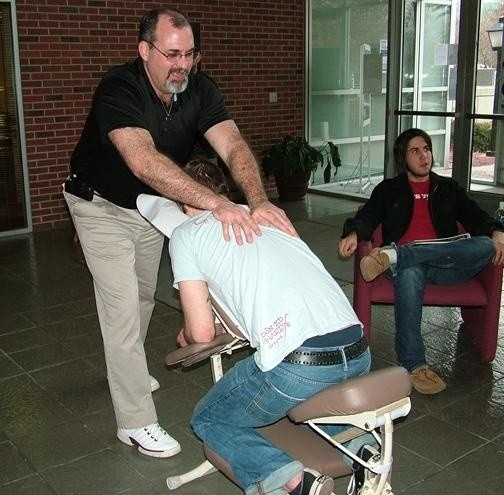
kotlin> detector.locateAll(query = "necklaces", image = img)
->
[158,94,174,121]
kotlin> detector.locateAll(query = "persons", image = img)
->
[169,157,383,495]
[61,8,298,459]
[338,129,504,395]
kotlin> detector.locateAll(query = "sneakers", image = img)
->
[288,467,335,495]
[360,247,390,282]
[148,374,161,391]
[409,364,446,395]
[118,422,181,457]
[347,444,382,494]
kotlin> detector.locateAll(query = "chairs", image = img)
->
[353,205,504,361]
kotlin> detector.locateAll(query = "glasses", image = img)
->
[146,39,196,61]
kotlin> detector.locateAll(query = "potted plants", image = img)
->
[258,133,341,201]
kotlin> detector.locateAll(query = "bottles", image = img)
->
[496,202,504,224]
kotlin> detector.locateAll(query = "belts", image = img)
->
[285,332,368,366]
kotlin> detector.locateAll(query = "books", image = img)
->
[411,233,471,245]
